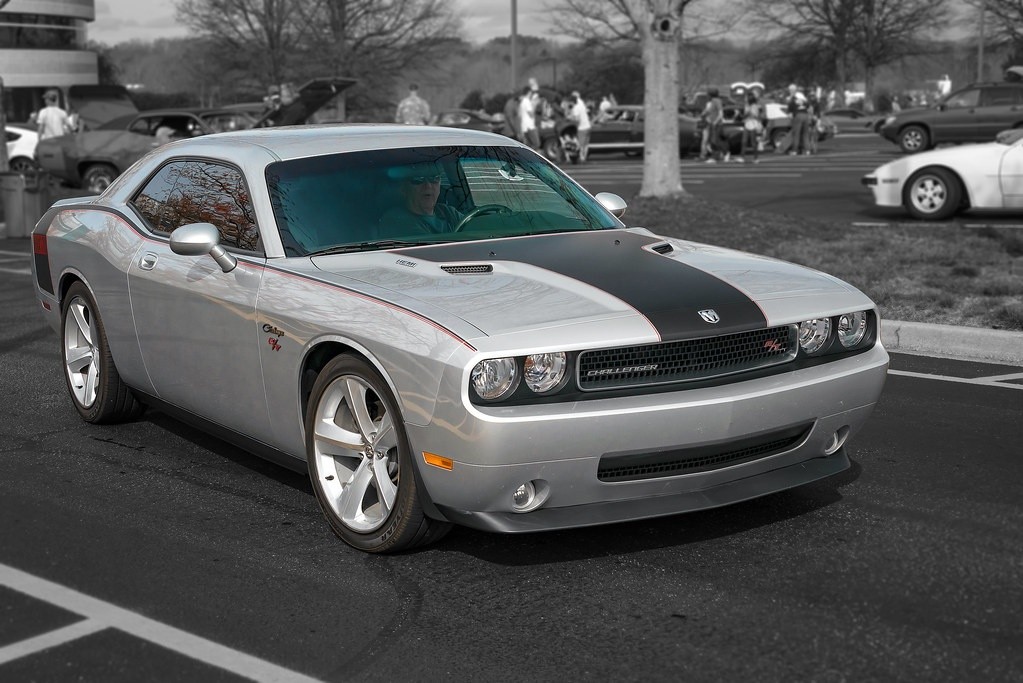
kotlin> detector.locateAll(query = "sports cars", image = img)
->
[30,122,891,556]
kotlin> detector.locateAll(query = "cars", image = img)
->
[31,77,359,196]
[427,103,841,159]
[876,83,1023,153]
[860,129,1022,218]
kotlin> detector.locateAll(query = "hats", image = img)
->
[43,91,57,99]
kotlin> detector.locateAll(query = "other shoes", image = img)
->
[722,151,731,162]
[734,157,747,164]
[705,157,717,163]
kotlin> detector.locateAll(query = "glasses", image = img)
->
[408,172,446,185]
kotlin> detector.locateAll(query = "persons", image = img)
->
[694,88,731,164]
[395,83,431,126]
[784,85,820,155]
[734,94,763,164]
[891,94,914,111]
[36,90,76,188]
[378,166,469,238]
[498,88,617,181]
[939,74,952,94]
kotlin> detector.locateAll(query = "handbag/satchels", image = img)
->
[696,114,708,130]
